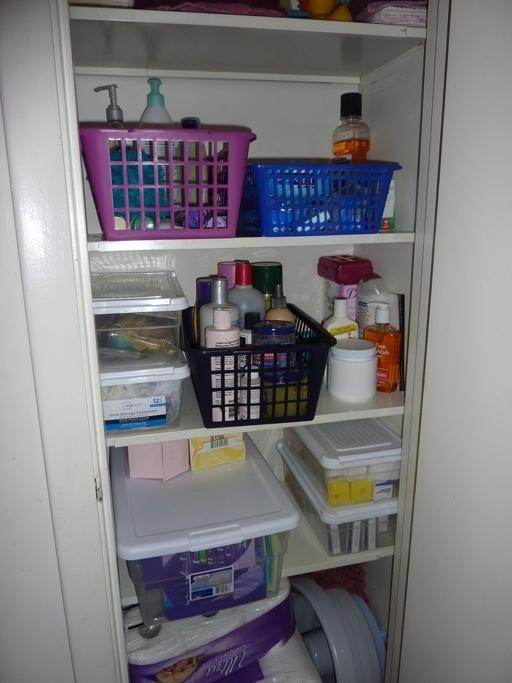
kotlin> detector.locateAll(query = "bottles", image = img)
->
[194,260,402,421]
[331,91,371,192]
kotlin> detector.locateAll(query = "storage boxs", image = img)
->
[273,439,395,555]
[97,346,191,429]
[110,428,302,632]
[90,270,191,373]
[288,417,404,509]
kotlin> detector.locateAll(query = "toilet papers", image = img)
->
[123,578,323,683]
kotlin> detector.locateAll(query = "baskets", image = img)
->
[180,303,337,428]
[214,161,402,237]
[79,127,257,241]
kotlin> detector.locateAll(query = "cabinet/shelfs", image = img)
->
[0,1,511,683]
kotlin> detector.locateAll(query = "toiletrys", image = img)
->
[92,86,135,156]
[136,78,181,211]
[192,257,310,422]
